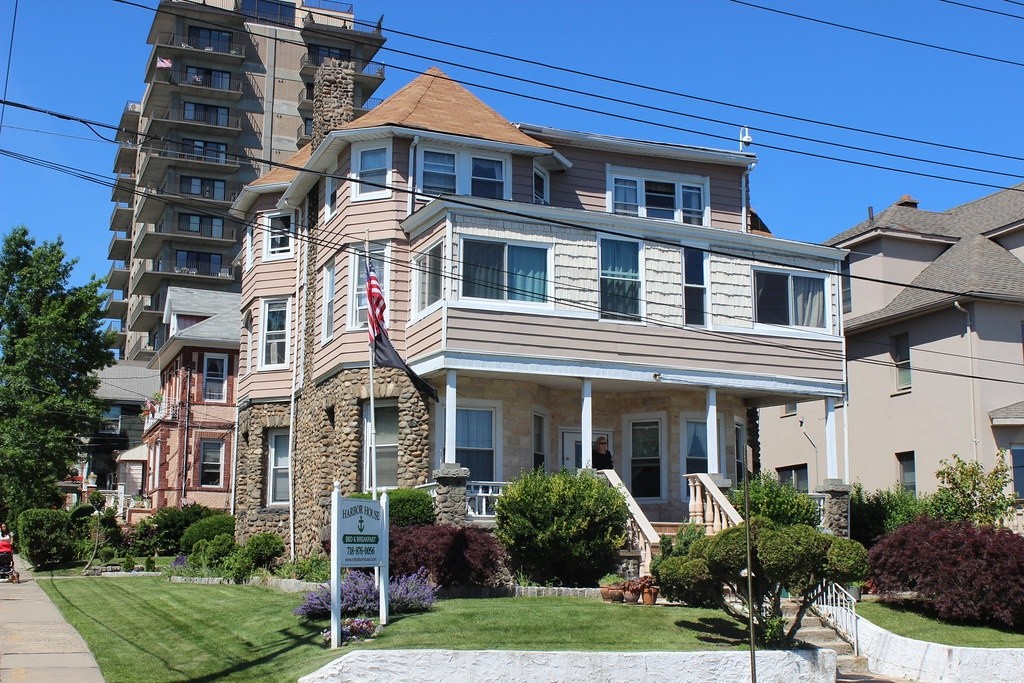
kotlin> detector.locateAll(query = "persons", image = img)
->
[0,523,14,545]
[592,437,614,471]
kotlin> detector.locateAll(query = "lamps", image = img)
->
[739,125,752,152]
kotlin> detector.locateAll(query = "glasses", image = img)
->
[600,442,607,445]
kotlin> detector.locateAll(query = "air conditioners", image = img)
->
[109,473,116,484]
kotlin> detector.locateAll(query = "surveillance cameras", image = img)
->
[741,135,752,146]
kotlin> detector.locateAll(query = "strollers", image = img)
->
[0,540,14,579]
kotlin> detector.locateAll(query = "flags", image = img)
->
[365,252,439,403]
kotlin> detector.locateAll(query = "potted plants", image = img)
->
[639,576,660,605]
[141,393,162,414]
[843,580,864,600]
[606,578,625,603]
[620,580,641,605]
[144,495,150,508]
[133,494,143,508]
[88,472,97,484]
[597,574,617,601]
